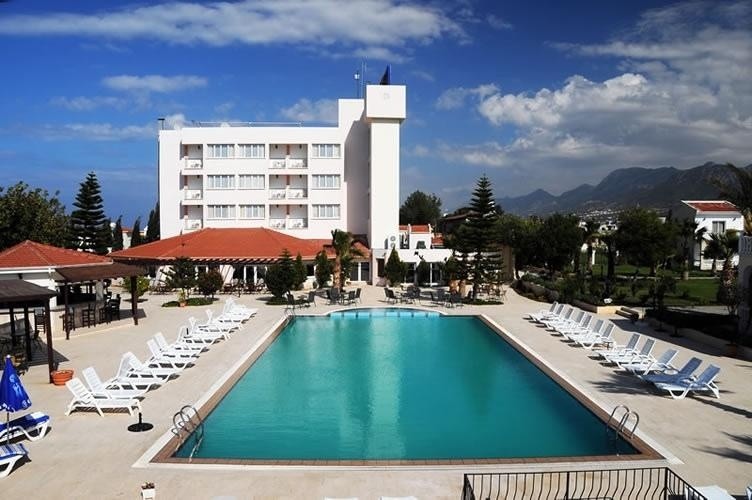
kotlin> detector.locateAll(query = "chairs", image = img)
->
[34,309,48,334]
[0,330,40,375]
[0,412,50,443]
[528,301,722,400]
[0,443,28,480]
[62,298,258,416]
[286,285,472,309]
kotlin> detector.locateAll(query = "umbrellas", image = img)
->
[0,354,32,444]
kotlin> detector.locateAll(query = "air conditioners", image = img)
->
[387,234,398,250]
